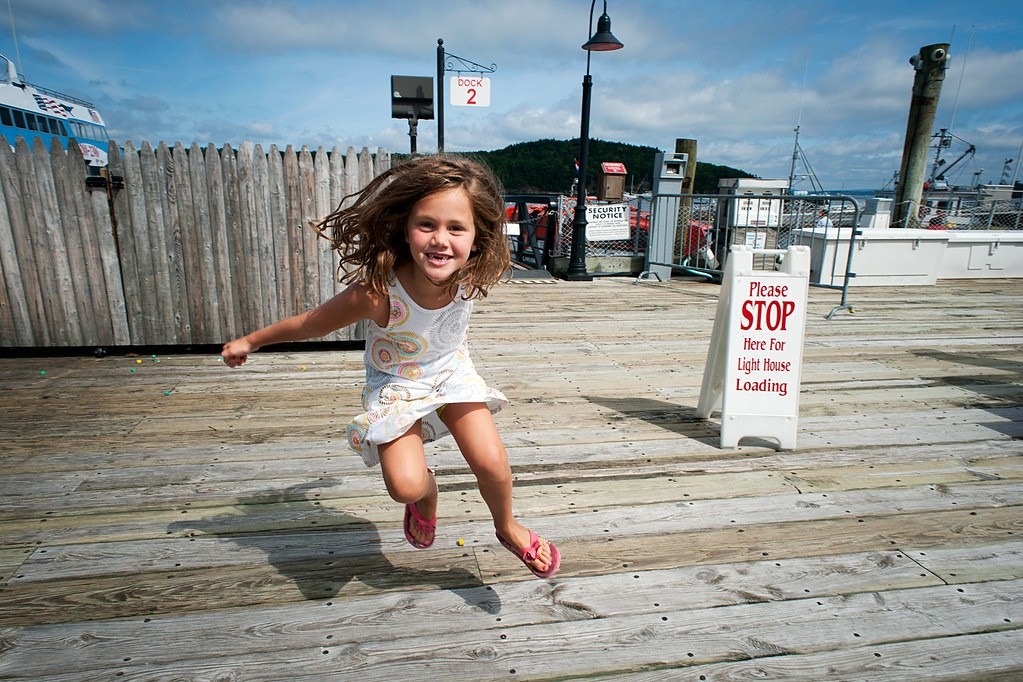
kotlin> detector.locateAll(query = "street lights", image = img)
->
[565,0,625,281]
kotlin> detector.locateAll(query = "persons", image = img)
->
[817,210,832,230]
[927,217,946,230]
[222,156,560,579]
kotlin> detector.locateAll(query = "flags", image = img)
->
[575,161,588,175]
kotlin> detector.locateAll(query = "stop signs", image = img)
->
[695,244,811,453]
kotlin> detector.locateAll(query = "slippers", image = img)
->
[403,468,438,548]
[495,528,561,579]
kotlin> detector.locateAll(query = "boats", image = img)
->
[635,191,653,199]
[0,51,134,198]
[624,193,636,200]
[503,202,715,258]
[694,198,714,203]
[876,128,982,228]
[692,60,865,227]
